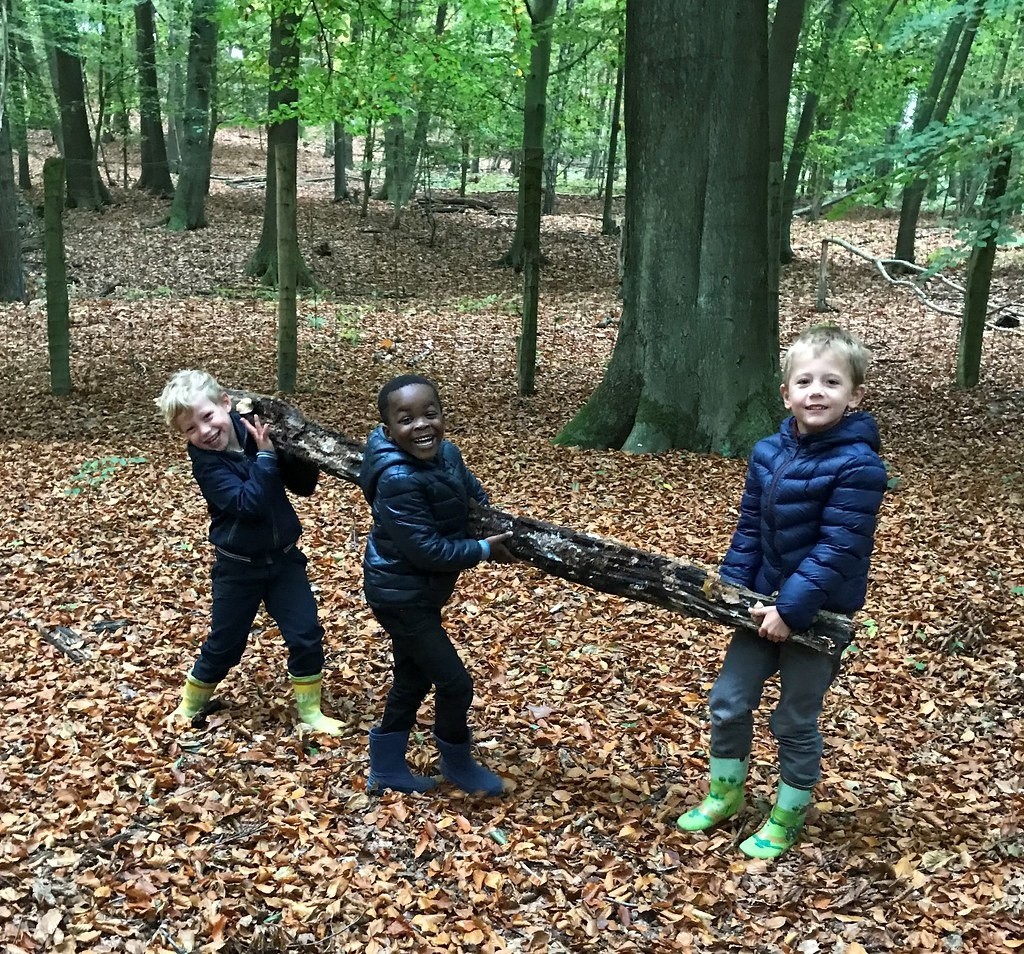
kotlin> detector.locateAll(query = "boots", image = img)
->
[287,671,347,738]
[158,669,218,728]
[432,733,502,798]
[368,728,437,794]
[737,777,813,862]
[677,753,752,836]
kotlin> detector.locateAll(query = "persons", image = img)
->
[678,326,887,860]
[360,374,513,797]
[158,369,348,737]
